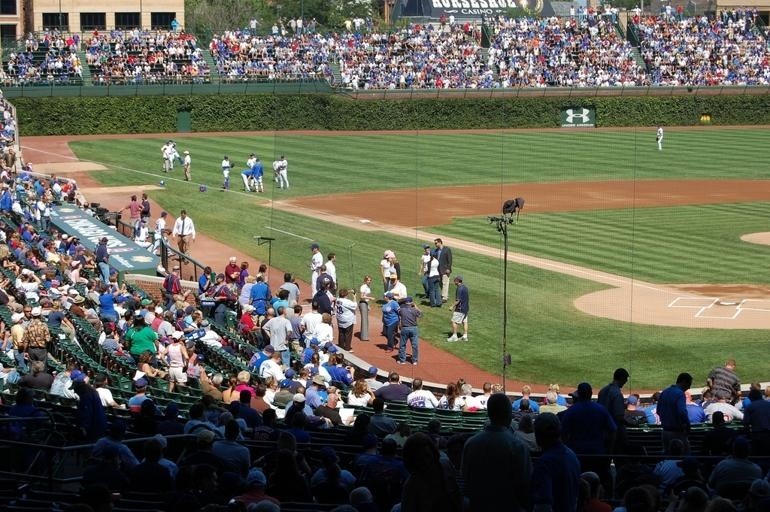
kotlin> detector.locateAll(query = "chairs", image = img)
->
[2,214,314,395]
[0,396,770,512]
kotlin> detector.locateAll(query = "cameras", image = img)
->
[352,287,357,295]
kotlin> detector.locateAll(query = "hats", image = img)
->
[369,368,378,373]
[308,244,319,248]
[142,299,184,340]
[424,244,429,249]
[351,487,373,506]
[247,469,266,488]
[294,393,306,402]
[385,291,393,298]
[11,289,86,323]
[68,378,85,390]
[265,338,338,389]
[406,298,413,304]
[455,276,462,280]
[135,379,148,388]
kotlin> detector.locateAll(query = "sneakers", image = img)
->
[460,337,468,342]
[447,337,459,343]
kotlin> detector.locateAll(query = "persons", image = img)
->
[119,194,151,235]
[181,151,191,181]
[209,17,337,86]
[84,28,211,87]
[140,210,197,253]
[1,101,19,219]
[445,276,469,342]
[2,366,770,512]
[18,171,76,218]
[656,125,664,152]
[1,27,84,86]
[380,249,422,366]
[240,153,263,193]
[334,1,769,88]
[2,218,346,394]
[336,276,375,354]
[273,154,290,190]
[82,202,96,218]
[161,140,184,173]
[222,154,234,188]
[420,237,452,308]
